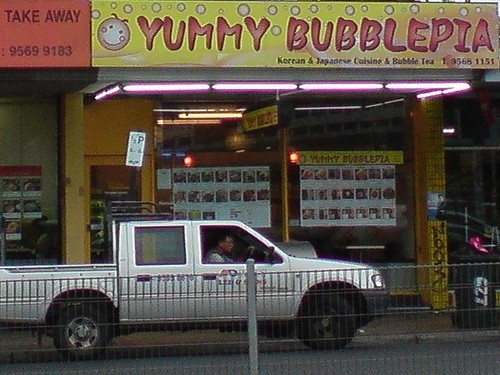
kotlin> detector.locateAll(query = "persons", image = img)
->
[204,233,235,262]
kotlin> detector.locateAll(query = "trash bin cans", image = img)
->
[449,250,500,329]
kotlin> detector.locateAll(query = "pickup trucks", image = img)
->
[0,219,388,359]
